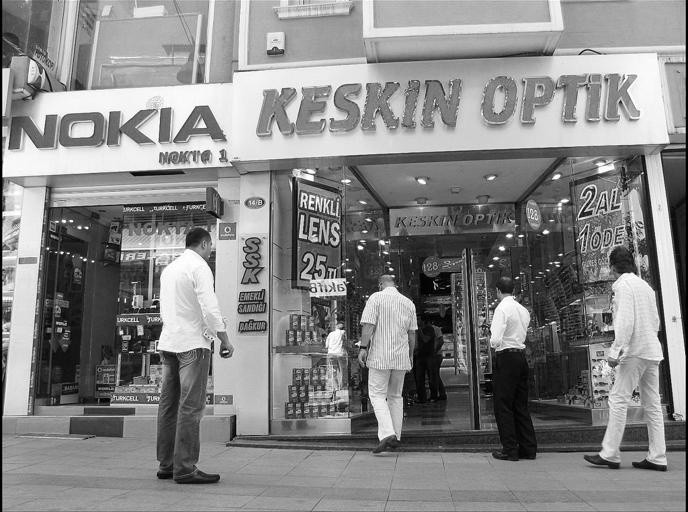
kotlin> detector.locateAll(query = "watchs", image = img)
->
[359,345,367,349]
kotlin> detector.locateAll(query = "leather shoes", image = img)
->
[584,453,619,469]
[631,459,668,472]
[640,460,641,461]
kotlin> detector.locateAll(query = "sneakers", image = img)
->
[157,470,174,480]
[373,435,401,453]
[493,449,536,461]
[175,470,221,485]
[414,393,449,403]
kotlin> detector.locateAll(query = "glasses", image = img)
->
[592,357,610,396]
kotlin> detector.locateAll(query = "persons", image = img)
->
[358,274,416,454]
[490,276,538,461]
[157,227,235,483]
[584,246,667,471]
[414,314,448,403]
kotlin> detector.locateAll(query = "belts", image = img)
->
[496,348,526,355]
